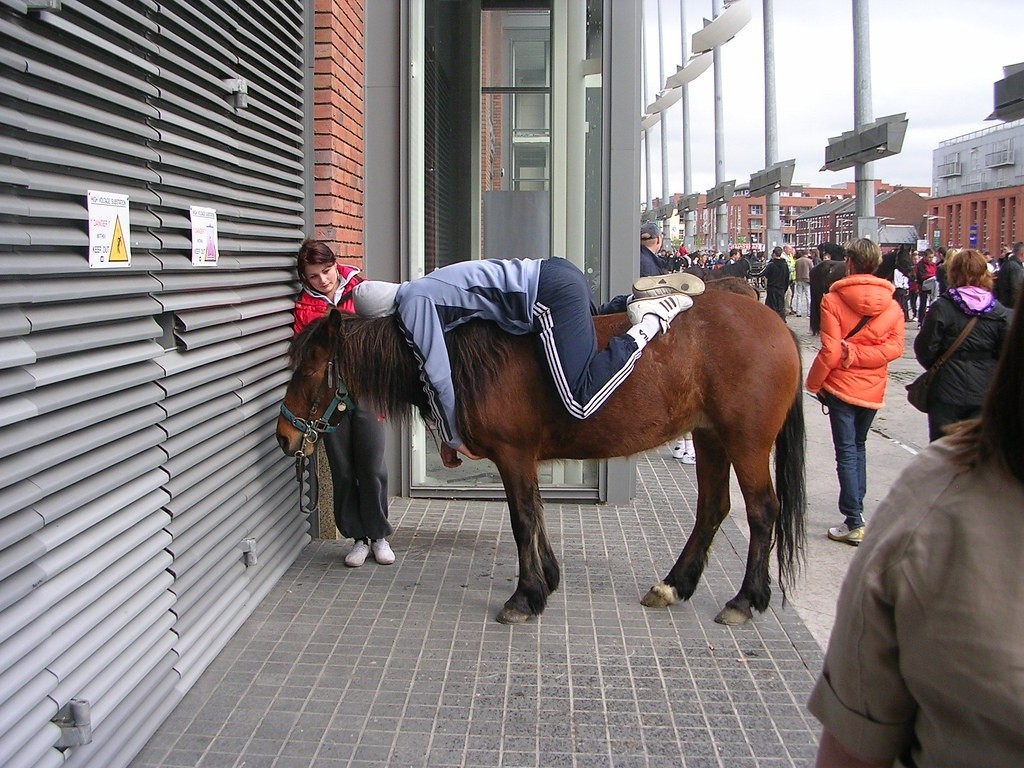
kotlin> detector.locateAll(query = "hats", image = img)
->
[641,222,662,240]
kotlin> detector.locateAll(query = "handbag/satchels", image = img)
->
[905,370,932,413]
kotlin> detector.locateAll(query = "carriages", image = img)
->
[682,253,761,282]
[672,251,700,272]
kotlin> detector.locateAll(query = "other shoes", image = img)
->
[917,325,922,330]
[907,319,913,322]
[788,310,797,316]
[912,316,918,321]
[796,315,802,318]
[806,316,810,318]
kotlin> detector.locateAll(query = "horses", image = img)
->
[683,249,754,282]
[276,278,807,628]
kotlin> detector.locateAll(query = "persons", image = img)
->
[806,286,1024,768]
[295,239,396,567]
[658,244,832,324]
[873,242,1024,330]
[353,256,704,468]
[913,248,1010,444]
[807,238,905,547]
[640,223,664,277]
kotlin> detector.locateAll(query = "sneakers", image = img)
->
[682,451,696,464]
[632,272,706,299]
[344,544,372,567]
[841,513,865,523]
[371,538,395,564]
[827,523,864,546]
[626,293,694,334]
[672,446,685,458]
